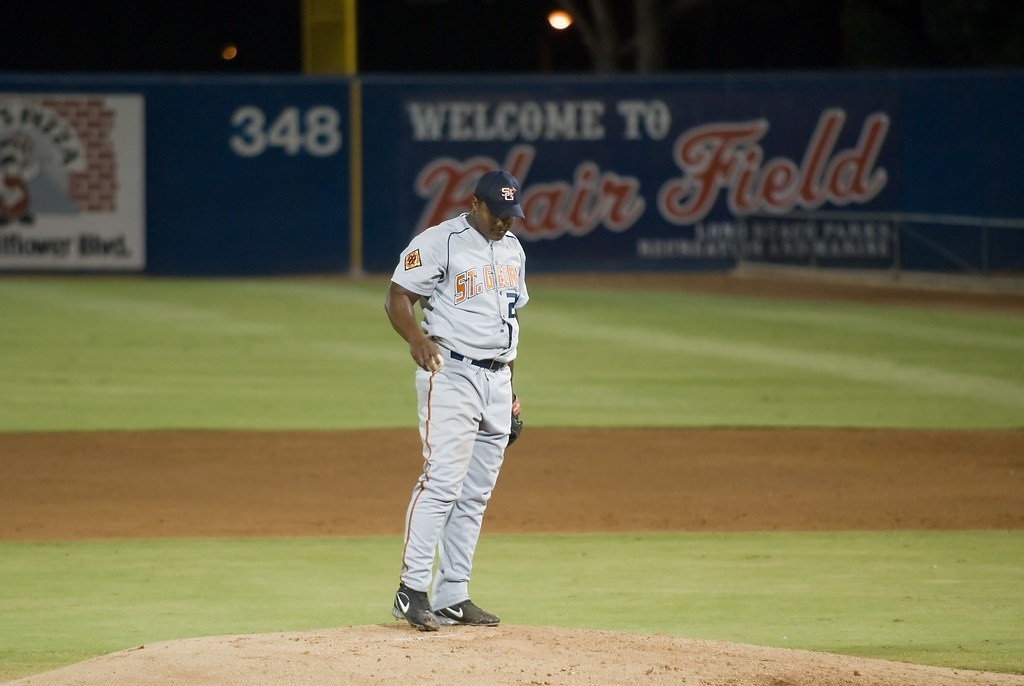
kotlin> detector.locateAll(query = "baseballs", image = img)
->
[426,354,444,372]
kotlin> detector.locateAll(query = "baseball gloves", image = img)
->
[506,396,525,446]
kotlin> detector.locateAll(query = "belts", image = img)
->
[450,349,508,371]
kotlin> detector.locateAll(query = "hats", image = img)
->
[474,170,527,220]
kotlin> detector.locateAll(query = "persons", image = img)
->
[385,171,530,631]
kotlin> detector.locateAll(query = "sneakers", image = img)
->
[391,583,439,631]
[433,598,500,625]
